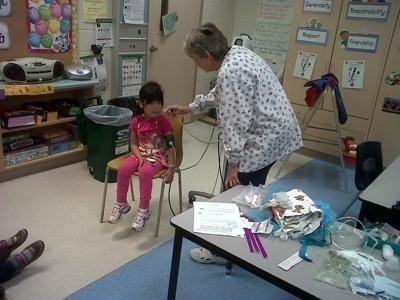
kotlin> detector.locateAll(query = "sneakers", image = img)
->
[7,229,28,256]
[107,200,131,224]
[18,240,45,271]
[130,208,151,231]
[190,247,228,265]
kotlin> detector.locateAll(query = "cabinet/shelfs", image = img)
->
[0,79,100,183]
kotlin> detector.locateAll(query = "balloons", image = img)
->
[29,8,41,23]
[63,3,72,19]
[61,18,70,34]
[47,17,59,35]
[35,21,46,35]
[49,2,62,18]
[41,33,52,47]
[23,31,40,49]
[39,5,51,19]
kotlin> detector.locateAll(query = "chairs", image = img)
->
[336,140,383,221]
[100,110,184,237]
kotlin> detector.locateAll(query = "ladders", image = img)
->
[274,84,349,192]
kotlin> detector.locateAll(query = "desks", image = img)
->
[167,183,400,300]
[357,154,400,229]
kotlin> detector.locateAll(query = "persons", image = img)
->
[108,83,176,232]
[1,227,44,282]
[166,21,304,264]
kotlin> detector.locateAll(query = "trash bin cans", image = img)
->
[84,105,133,183]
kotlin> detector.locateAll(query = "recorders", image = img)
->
[0,56,66,84]
